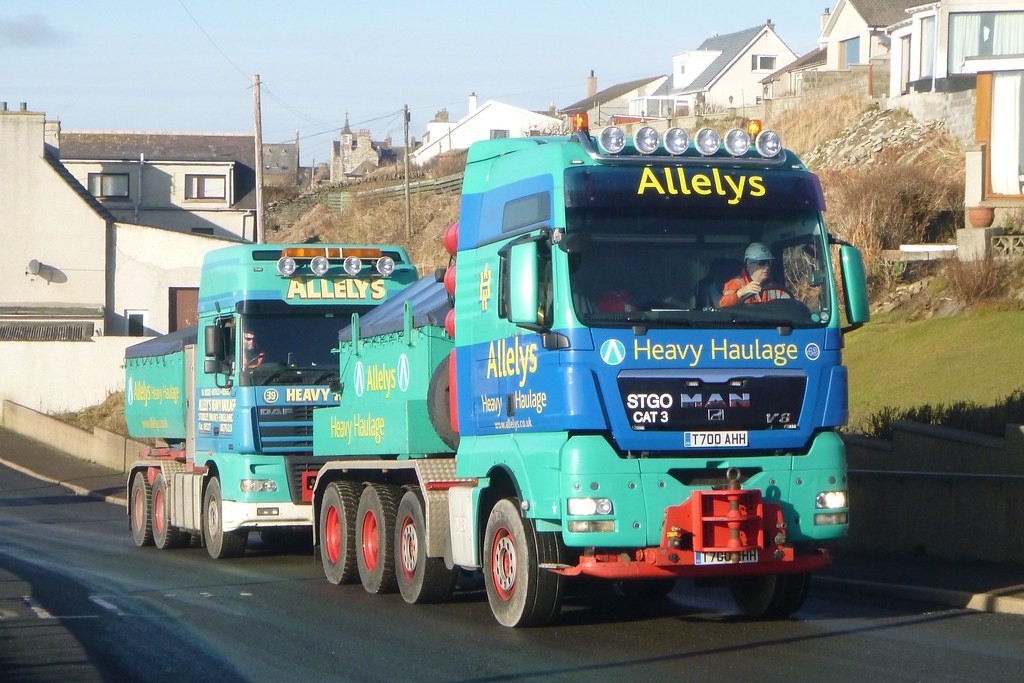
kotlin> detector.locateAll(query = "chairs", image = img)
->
[695,257,743,310]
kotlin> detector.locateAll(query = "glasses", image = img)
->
[244,336,256,341]
[749,260,772,266]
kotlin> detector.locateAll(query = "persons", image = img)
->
[223,330,265,368]
[719,243,794,306]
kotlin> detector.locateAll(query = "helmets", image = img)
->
[744,243,774,262]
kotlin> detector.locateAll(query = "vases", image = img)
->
[967,207,996,227]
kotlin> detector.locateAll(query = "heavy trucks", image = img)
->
[123,243,418,559]
[311,125,871,629]
